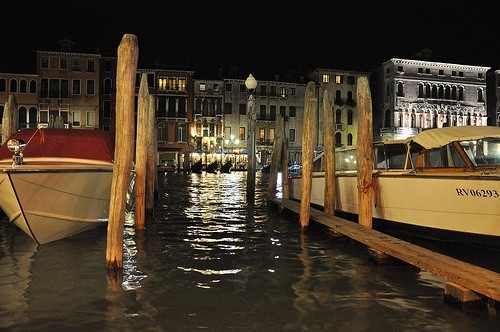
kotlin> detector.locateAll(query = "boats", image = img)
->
[290,126,500,237]
[0,126,116,246]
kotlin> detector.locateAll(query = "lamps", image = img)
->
[7,138,26,165]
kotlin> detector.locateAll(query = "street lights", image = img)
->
[243,73,258,190]
